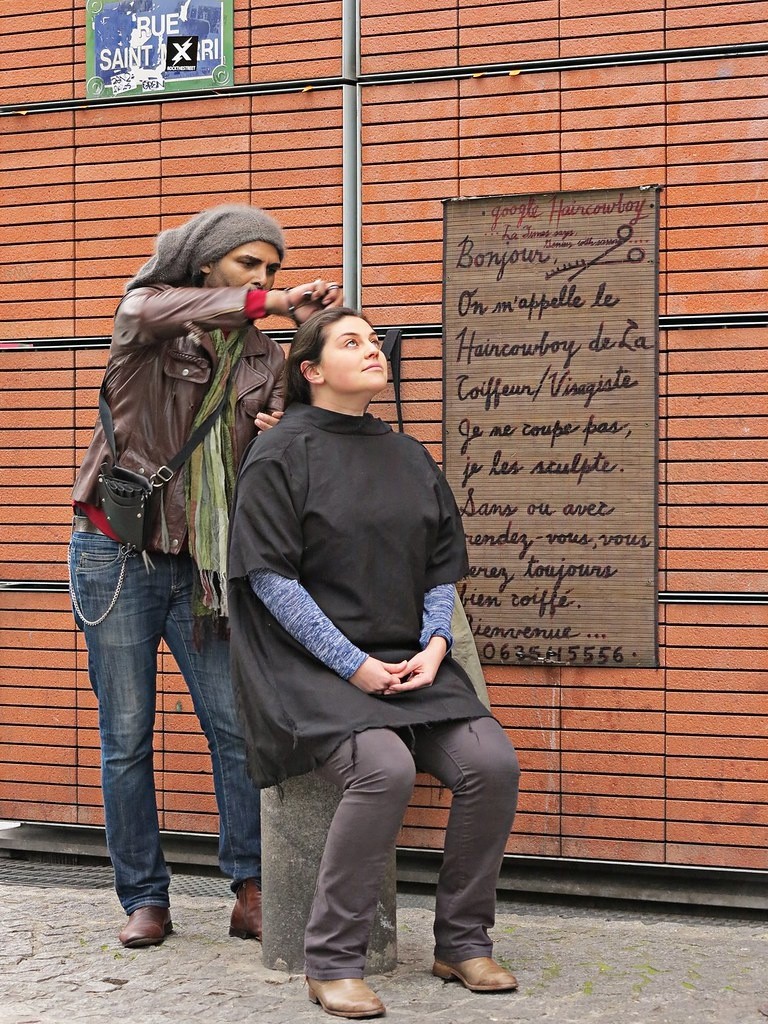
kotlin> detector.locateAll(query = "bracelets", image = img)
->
[284,286,301,328]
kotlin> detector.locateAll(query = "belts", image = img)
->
[73,516,106,536]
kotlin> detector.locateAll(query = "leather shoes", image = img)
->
[305,975,385,1019]
[228,879,264,943]
[119,904,173,948]
[433,955,519,991]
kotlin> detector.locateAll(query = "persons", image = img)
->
[226,307,520,1015]
[66,200,347,953]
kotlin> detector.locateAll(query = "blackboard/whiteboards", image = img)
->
[437,183,662,672]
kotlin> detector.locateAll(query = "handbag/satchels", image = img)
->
[96,461,155,553]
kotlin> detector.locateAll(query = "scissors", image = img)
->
[302,284,340,312]
